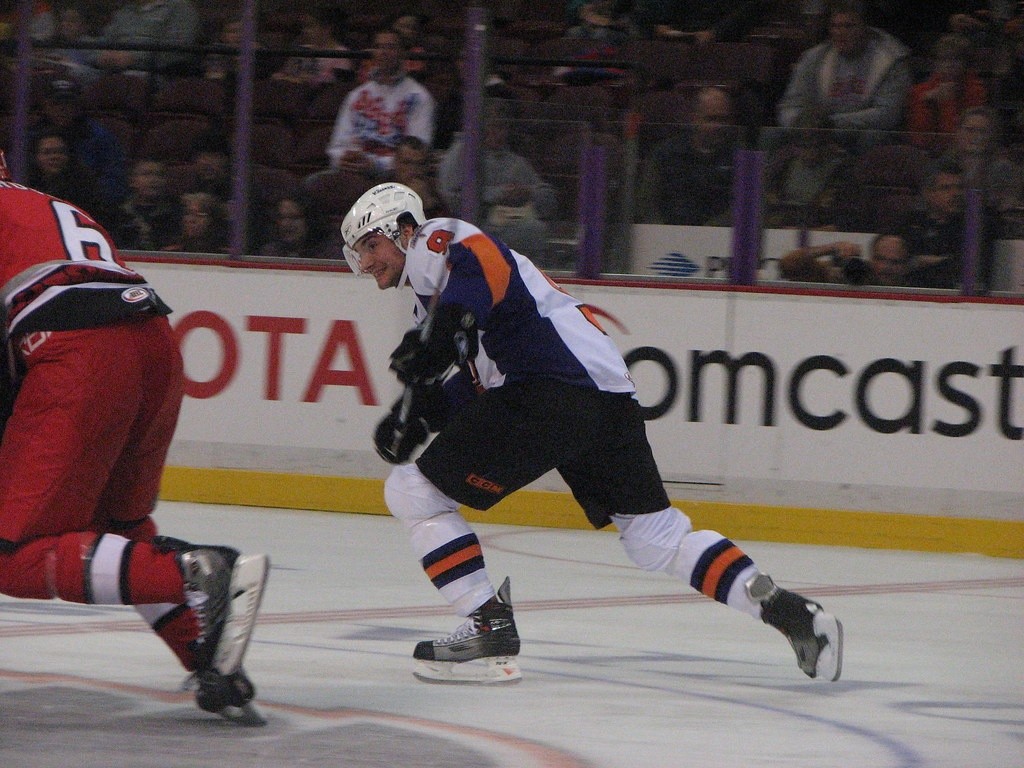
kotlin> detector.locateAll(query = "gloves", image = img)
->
[374,381,447,465]
[388,301,481,385]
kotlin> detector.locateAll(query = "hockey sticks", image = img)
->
[387,139,469,458]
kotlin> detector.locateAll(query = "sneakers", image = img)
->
[153,534,268,680]
[745,574,844,683]
[413,577,523,681]
[186,641,268,728]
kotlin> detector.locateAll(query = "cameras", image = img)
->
[834,253,869,285]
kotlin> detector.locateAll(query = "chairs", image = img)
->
[0,0,1024,292]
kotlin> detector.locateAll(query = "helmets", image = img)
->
[341,180,428,249]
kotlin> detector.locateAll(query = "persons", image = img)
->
[339,182,843,682]
[0,150,269,729]
[0,0,1024,291]
